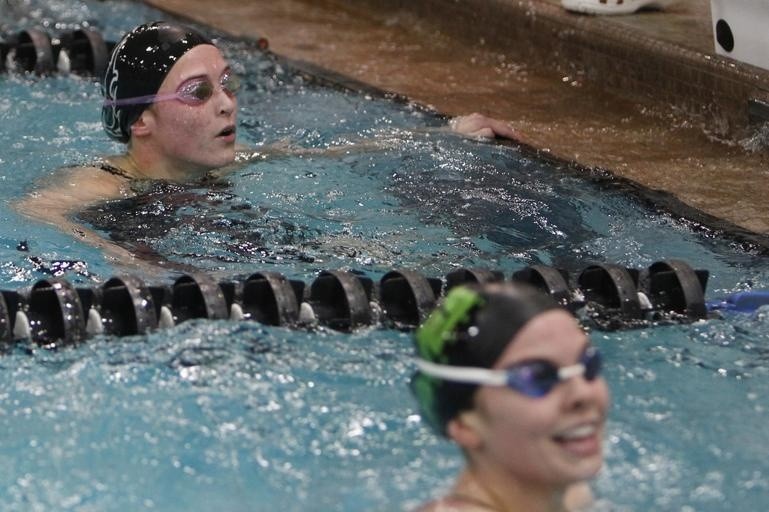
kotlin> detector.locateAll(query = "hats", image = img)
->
[99,22,225,144]
[411,279,582,437]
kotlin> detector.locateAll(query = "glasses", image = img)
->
[141,67,244,110]
[440,344,603,399]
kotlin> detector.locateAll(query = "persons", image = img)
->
[408,281,610,512]
[10,21,538,274]
